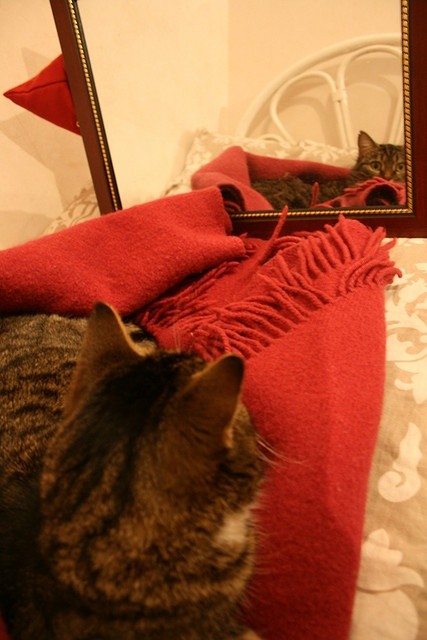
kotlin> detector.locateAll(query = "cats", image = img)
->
[0,299,313,640]
[250,130,405,208]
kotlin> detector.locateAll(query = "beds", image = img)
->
[162,33,400,211]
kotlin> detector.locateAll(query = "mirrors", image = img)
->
[49,0,427,240]
